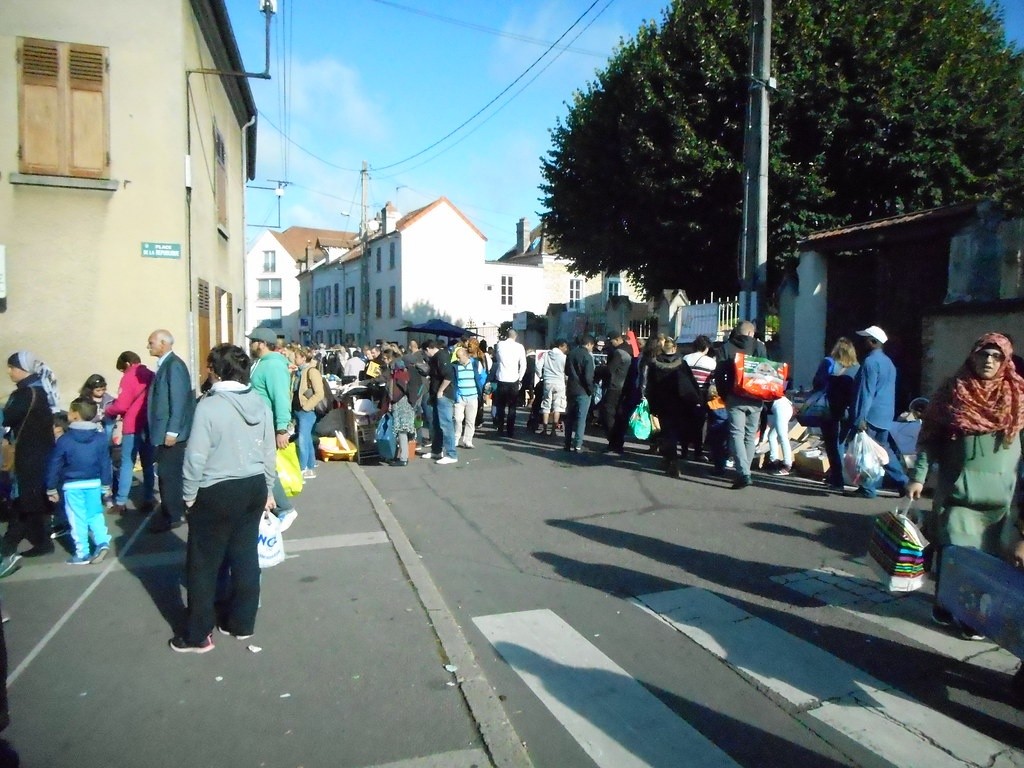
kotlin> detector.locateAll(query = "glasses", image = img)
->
[976,351,1002,361]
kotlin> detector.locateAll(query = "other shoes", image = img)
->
[931,606,952,624]
[732,475,753,489]
[218,624,253,640]
[170,633,214,654]
[959,619,985,640]
[390,424,711,467]
[0,503,298,578]
[711,467,727,475]
[773,469,792,476]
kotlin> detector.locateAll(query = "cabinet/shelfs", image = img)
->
[342,395,380,466]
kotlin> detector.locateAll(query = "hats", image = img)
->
[857,327,887,344]
[245,328,277,344]
[607,330,621,340]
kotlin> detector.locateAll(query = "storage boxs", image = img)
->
[789,394,833,479]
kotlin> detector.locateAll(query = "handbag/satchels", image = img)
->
[374,411,397,459]
[258,510,284,568]
[628,398,662,440]
[865,497,931,592]
[366,361,381,378]
[303,367,334,413]
[0,439,15,471]
[844,431,890,486]
[276,442,302,497]
[732,352,788,400]
[796,390,831,427]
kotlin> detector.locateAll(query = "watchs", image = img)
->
[276,429,288,435]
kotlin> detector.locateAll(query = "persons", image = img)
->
[77,375,116,423]
[105,351,156,515]
[46,395,113,566]
[1000,427,1024,565]
[2,351,55,558]
[842,325,911,498]
[147,329,195,534]
[909,398,930,419]
[181,343,276,653]
[812,337,863,492]
[906,332,1024,641]
[245,320,794,533]
[0,407,28,578]
